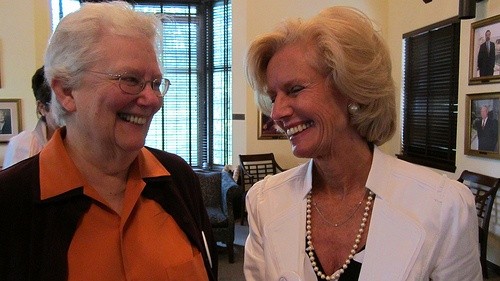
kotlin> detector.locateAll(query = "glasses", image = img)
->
[82,69,171,97]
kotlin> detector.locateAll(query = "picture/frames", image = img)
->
[0,98,22,144]
[464,92,500,160]
[257,109,289,140]
[467,14,500,86]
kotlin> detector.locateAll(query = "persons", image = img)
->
[0,4,218,281]
[244,6,486,281]
[477,29,497,150]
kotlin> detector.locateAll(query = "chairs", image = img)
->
[196,153,288,263]
[456,170,500,279]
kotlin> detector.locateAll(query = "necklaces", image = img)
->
[307,190,373,281]
[312,185,366,227]
